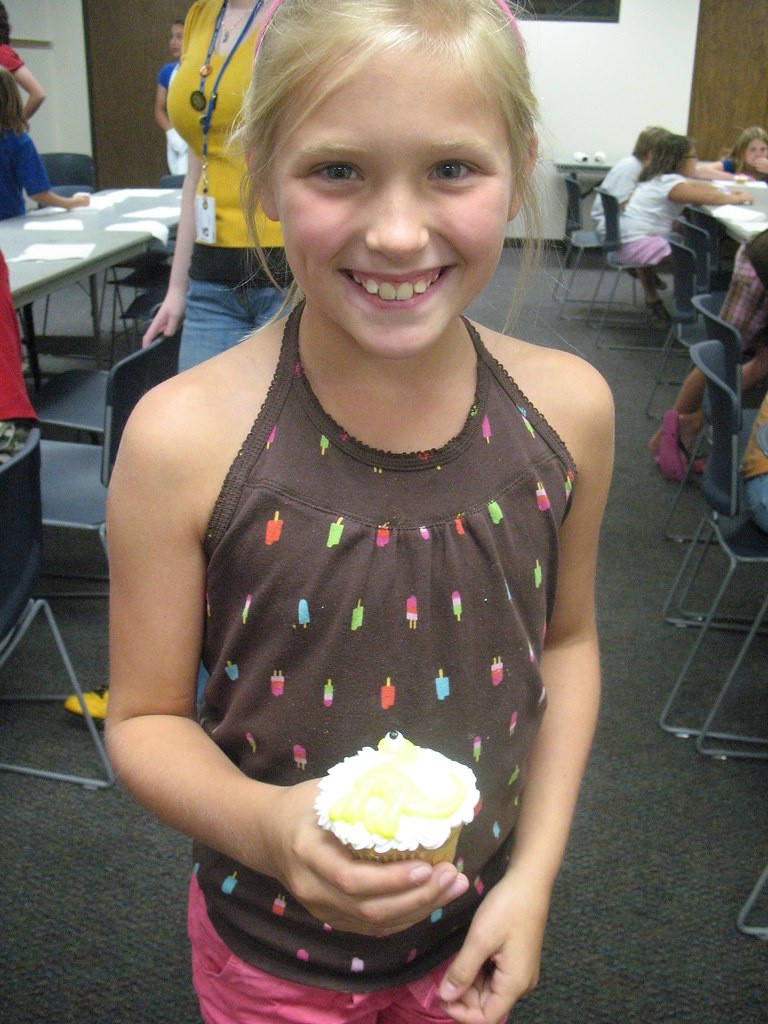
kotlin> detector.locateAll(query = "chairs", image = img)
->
[0,151,768,936]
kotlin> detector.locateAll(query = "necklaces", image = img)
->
[222,0,258,43]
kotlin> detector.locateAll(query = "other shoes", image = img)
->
[63,687,109,721]
[650,411,709,480]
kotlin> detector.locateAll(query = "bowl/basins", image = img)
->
[339,823,461,864]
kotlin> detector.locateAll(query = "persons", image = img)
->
[591,126,768,324]
[154,18,189,175]
[648,229,768,533]
[0,250,40,468]
[64,0,303,717]
[0,1,90,383]
[103,0,616,1024]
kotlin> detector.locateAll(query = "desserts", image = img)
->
[313,730,481,866]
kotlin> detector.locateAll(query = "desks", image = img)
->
[553,156,617,246]
[0,216,158,394]
[30,188,183,356]
[683,178,768,289]
